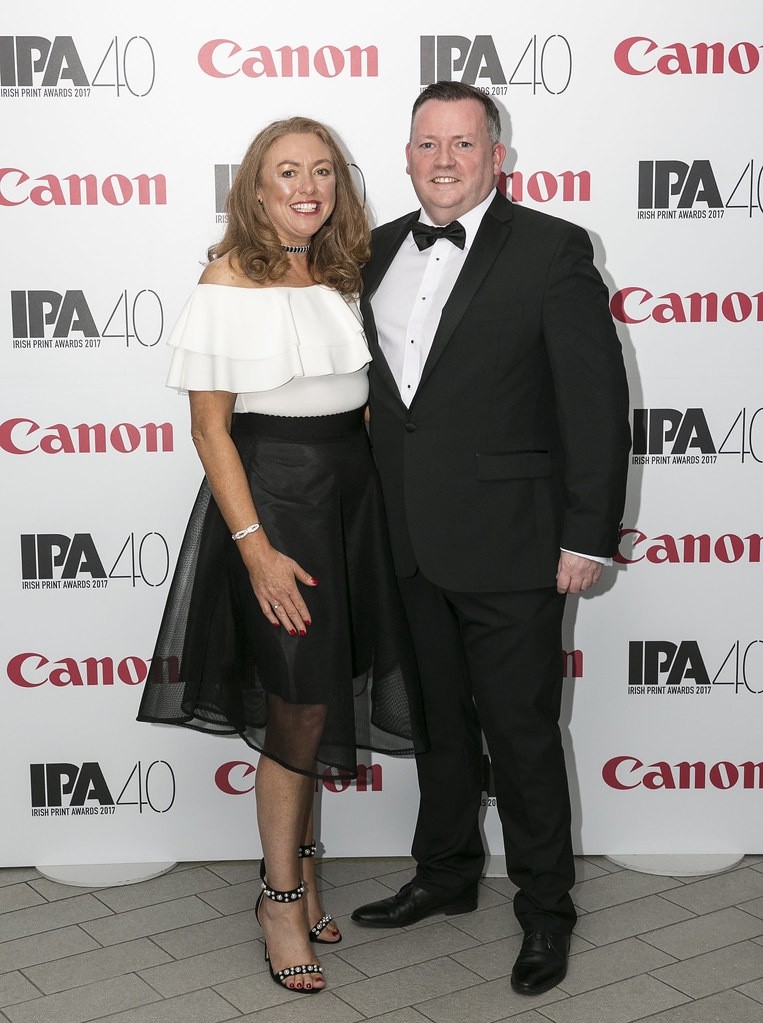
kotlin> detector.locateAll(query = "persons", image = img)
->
[350,82,632,996]
[138,116,430,993]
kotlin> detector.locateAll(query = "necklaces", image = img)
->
[280,244,311,253]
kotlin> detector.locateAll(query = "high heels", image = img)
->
[254,857,325,994]
[297,839,342,944]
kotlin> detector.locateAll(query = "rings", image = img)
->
[272,604,282,608]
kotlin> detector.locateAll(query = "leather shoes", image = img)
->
[510,928,570,995]
[350,881,478,928]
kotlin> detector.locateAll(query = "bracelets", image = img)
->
[232,520,263,540]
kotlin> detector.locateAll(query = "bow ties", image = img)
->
[410,219,466,251]
